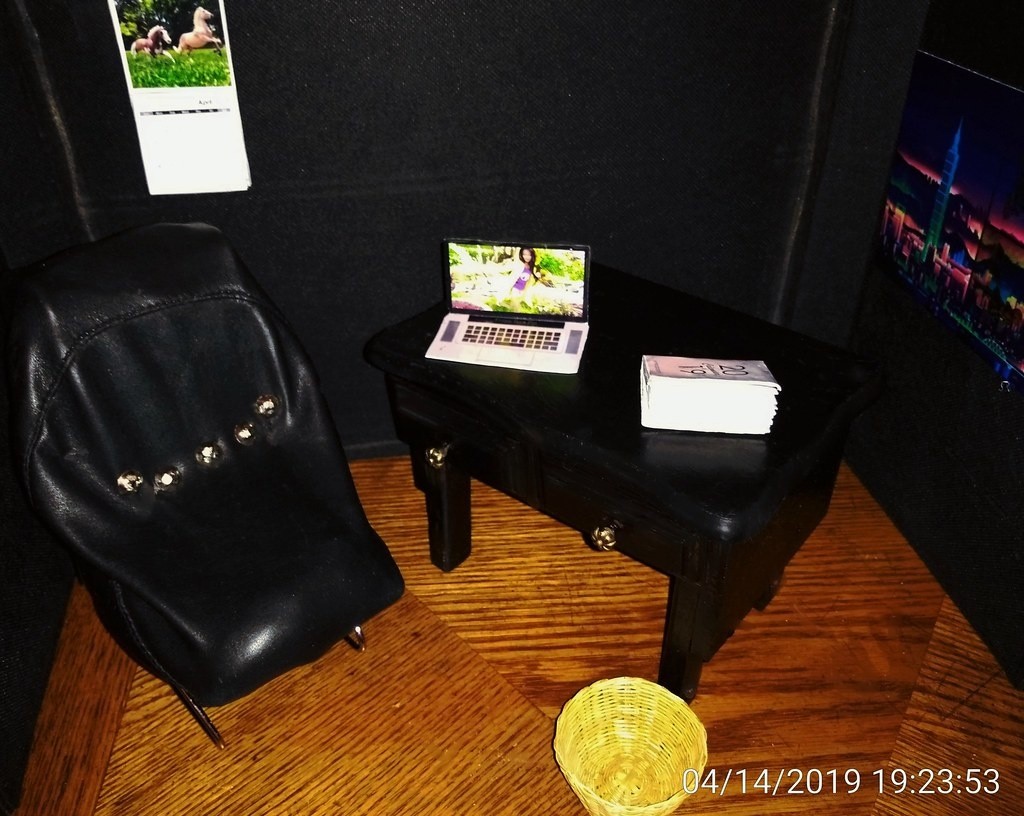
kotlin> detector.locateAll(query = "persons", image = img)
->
[490,248,539,307]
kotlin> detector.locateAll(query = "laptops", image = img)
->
[424,237,592,375]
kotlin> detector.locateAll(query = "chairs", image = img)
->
[1,225,403,747]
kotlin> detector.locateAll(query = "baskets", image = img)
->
[555,677,709,816]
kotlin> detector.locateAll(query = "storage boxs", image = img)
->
[554,675,707,814]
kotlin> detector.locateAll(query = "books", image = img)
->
[639,355,782,434]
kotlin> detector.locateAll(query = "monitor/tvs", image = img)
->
[873,46,1023,392]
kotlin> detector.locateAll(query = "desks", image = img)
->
[365,259,848,701]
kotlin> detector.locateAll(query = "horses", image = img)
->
[173,7,224,57]
[130,25,175,64]
